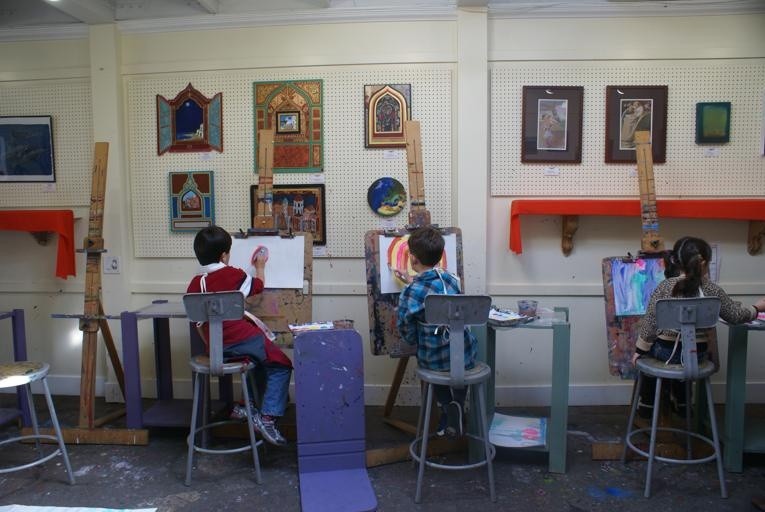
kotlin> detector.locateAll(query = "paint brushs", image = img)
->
[394,269,408,282]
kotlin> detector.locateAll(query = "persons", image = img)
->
[185,226,294,447]
[396,226,478,437]
[631,236,765,435]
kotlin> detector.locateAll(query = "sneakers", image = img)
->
[671,398,694,419]
[435,414,447,437]
[639,402,653,419]
[447,401,463,437]
[250,412,288,447]
[230,403,258,421]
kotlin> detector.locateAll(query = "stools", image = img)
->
[0,360,83,487]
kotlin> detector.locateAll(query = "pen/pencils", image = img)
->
[254,250,262,261]
[524,316,540,323]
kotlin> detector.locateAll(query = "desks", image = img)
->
[698,312,765,475]
[120,299,203,442]
[446,303,572,475]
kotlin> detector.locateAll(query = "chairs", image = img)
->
[183,290,274,485]
[407,293,500,502]
[620,296,729,502]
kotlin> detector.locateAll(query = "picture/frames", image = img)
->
[361,84,412,148]
[252,79,325,173]
[695,102,731,143]
[0,115,56,183]
[521,84,584,164]
[250,184,327,247]
[605,84,667,165]
